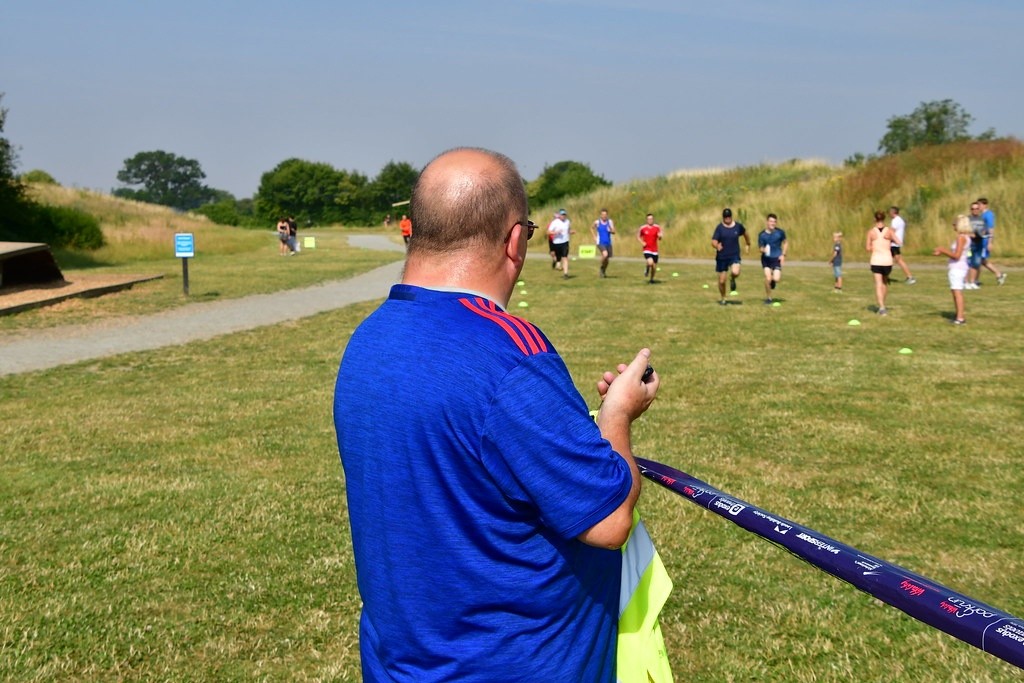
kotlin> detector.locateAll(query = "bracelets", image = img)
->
[782,254,786,257]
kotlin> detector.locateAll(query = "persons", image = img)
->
[712,209,751,305]
[384,214,391,226]
[758,214,788,304]
[829,232,844,289]
[888,207,917,284]
[399,215,412,247]
[590,208,616,277]
[546,208,577,277]
[277,215,297,255]
[637,213,663,283]
[977,198,1008,285]
[932,214,973,325]
[865,210,902,317]
[334,146,659,683]
[964,202,987,289]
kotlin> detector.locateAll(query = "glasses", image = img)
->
[971,208,979,210]
[503,220,539,243]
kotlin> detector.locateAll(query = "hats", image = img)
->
[559,211,567,215]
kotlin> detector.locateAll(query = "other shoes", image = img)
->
[645,268,650,276]
[771,280,776,288]
[996,273,1008,284]
[729,278,735,290]
[564,273,568,279]
[720,299,726,305]
[765,299,772,303]
[875,307,887,315]
[906,277,916,284]
[650,280,654,284]
[964,282,980,289]
[600,270,606,277]
[952,318,967,324]
